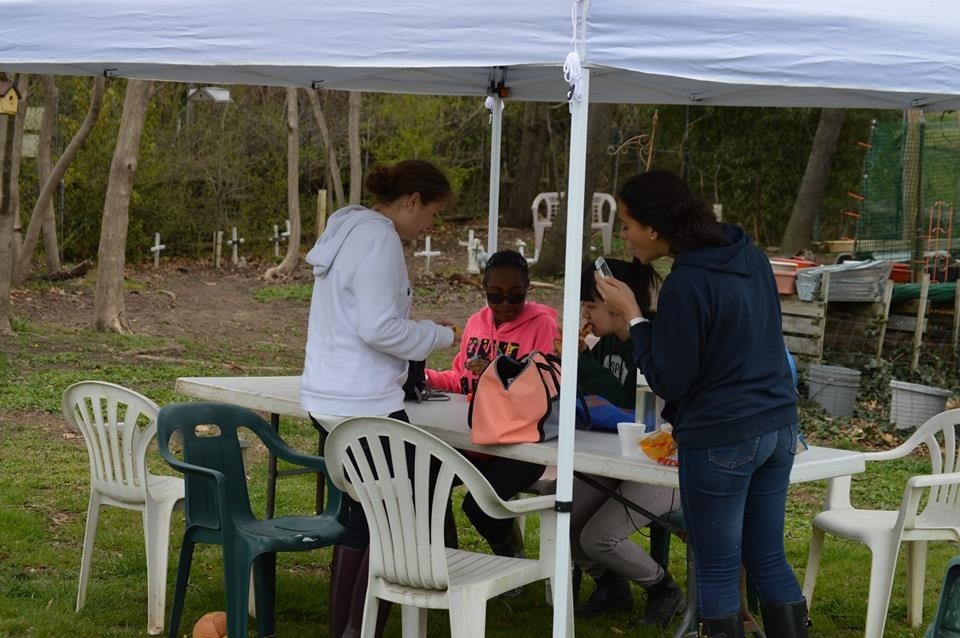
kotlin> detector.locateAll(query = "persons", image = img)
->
[560,256,684,632]
[301,159,463,638]
[425,248,561,598]
[594,167,810,638]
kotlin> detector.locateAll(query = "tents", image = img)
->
[1,1,960,638]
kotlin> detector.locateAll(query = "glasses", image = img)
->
[485,283,526,304]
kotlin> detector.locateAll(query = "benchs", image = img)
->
[532,191,617,264]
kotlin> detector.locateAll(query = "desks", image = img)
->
[173,373,869,638]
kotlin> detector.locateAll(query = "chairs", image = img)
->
[63,381,258,636]
[802,407,959,638]
[323,415,554,637]
[156,401,344,635]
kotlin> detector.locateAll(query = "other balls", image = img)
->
[191,611,258,638]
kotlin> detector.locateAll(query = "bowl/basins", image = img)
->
[770,257,819,295]
[889,263,915,283]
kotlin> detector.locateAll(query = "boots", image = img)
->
[574,581,634,617]
[760,596,813,638]
[697,607,745,638]
[328,545,364,638]
[341,544,392,638]
[641,569,687,630]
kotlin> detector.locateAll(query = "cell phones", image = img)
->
[595,257,612,278]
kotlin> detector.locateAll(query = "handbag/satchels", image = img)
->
[467,350,593,446]
[402,359,431,402]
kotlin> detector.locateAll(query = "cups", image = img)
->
[617,422,646,455]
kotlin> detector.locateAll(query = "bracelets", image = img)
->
[627,317,649,331]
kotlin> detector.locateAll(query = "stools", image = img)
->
[648,508,759,615]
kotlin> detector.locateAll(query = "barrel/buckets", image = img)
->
[807,364,861,419]
[888,379,953,430]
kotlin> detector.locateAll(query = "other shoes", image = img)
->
[499,587,522,597]
